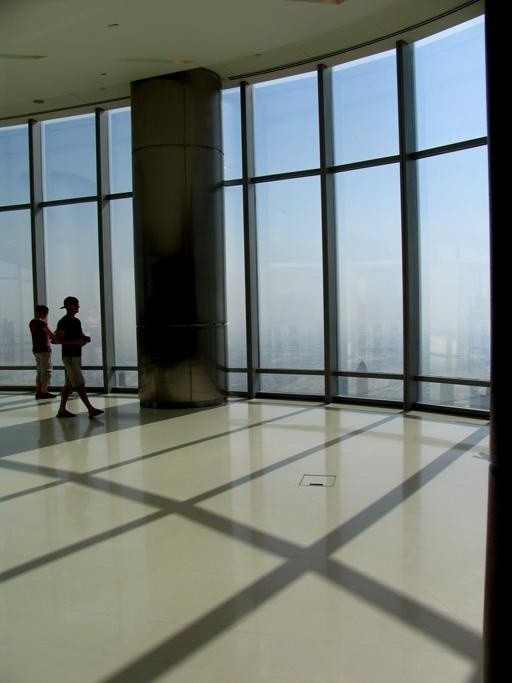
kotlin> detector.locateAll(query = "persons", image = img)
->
[28,304,58,399]
[49,295,105,419]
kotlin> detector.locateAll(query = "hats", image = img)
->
[59,296,80,310]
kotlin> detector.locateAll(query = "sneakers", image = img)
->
[35,390,56,399]
[56,408,104,419]
